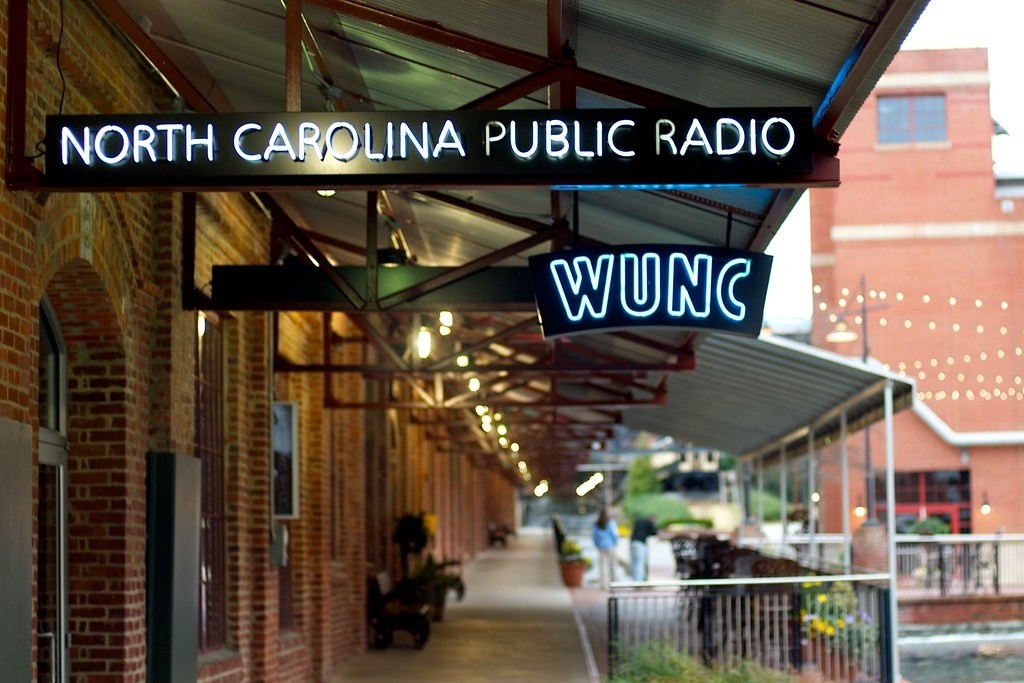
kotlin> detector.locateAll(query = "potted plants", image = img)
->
[561,561,585,587]
[392,551,466,622]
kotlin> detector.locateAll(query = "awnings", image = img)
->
[623,327,916,681]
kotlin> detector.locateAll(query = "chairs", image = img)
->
[672,534,804,671]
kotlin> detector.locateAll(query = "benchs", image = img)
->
[368,570,432,651]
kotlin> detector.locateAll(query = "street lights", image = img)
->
[824,275,891,571]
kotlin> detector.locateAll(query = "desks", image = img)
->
[711,572,767,660]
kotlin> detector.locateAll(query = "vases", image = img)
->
[799,638,862,683]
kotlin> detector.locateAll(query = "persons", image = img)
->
[630,514,654,588]
[593,505,619,581]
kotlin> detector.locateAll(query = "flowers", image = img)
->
[797,573,880,656]
[557,540,593,568]
